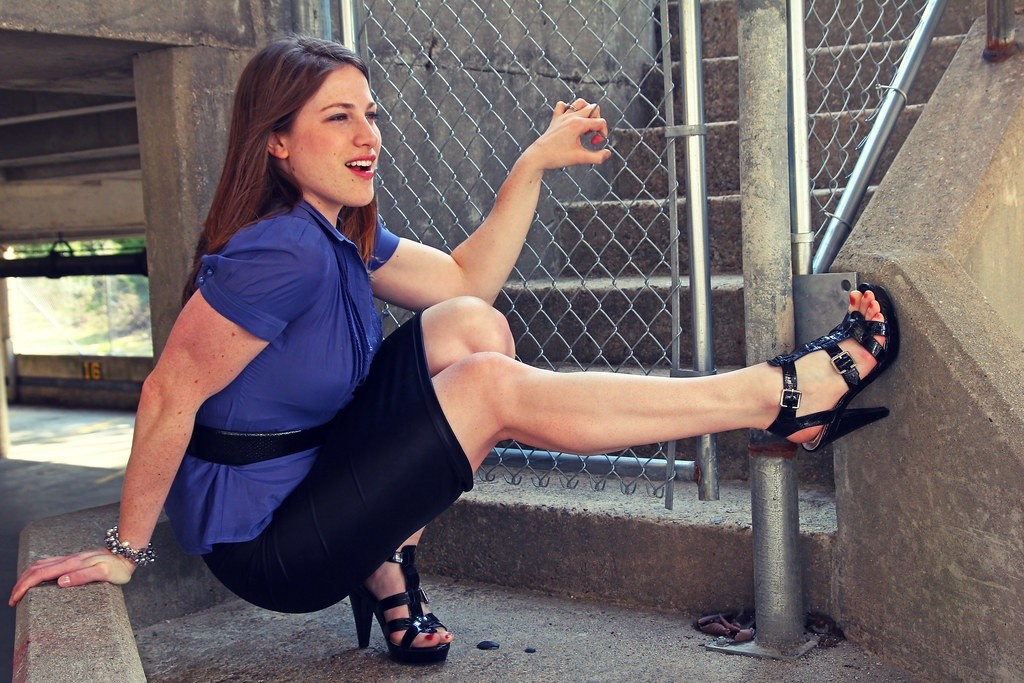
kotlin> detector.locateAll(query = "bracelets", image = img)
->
[104,525,157,567]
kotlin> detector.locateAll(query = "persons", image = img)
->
[9,34,901,665]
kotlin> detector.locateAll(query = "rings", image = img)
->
[569,105,577,112]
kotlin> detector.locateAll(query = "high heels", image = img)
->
[348,545,450,665]
[765,282,900,453]
